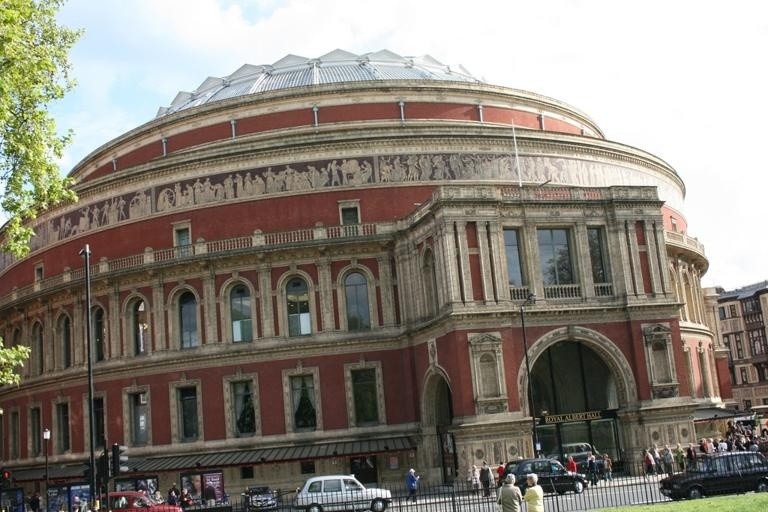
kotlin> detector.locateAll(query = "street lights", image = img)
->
[79,243,101,508]
[520,292,542,458]
[42,427,51,511]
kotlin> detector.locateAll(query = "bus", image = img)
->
[748,404,768,437]
[734,415,764,430]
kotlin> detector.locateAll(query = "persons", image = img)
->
[524,472,544,512]
[206,480,217,509]
[496,473,523,512]
[405,468,420,502]
[0,481,194,512]
[471,420,767,497]
[189,476,201,497]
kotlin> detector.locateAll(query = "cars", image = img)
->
[499,456,585,497]
[291,473,394,511]
[659,451,768,500]
[242,485,278,508]
[92,491,184,511]
[548,441,602,462]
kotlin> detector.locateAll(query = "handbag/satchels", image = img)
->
[494,504,503,512]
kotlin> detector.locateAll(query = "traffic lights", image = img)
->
[0,471,12,489]
[119,446,128,474]
[83,461,90,475]
